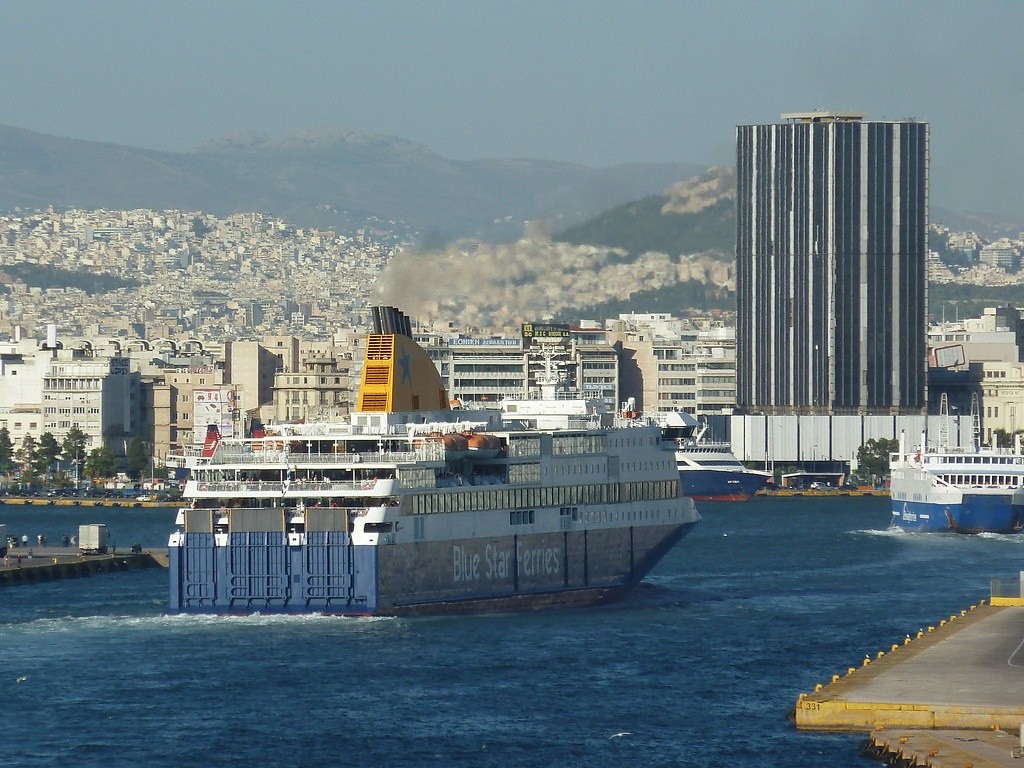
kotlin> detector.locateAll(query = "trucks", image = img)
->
[79,524,110,555]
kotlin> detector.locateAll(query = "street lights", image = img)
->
[813,445,818,473]
[840,456,842,473]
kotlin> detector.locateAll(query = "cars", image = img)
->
[136,495,152,501]
[811,482,826,489]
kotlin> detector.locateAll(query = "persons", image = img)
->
[314,475,318,481]
[43,536,47,548]
[313,502,319,507]
[381,499,398,507]
[220,501,226,518]
[227,499,243,508]
[16,551,25,568]
[332,502,337,507]
[62,534,70,547]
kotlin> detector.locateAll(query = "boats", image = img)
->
[889,429,1024,534]
[167,306,702,617]
[611,397,775,501]
